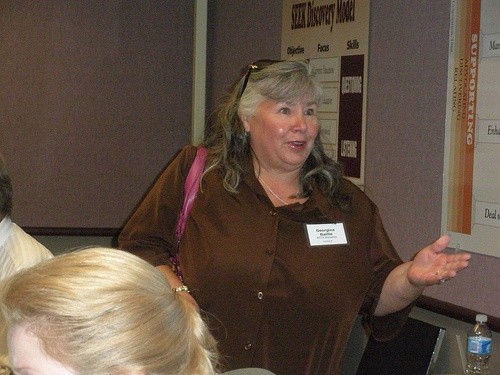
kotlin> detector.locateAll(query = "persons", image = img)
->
[0,152,224,375]
[118,57,470,375]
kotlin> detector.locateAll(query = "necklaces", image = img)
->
[253,168,300,205]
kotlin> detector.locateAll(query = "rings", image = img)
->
[436,270,445,283]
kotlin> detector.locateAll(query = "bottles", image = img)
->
[466,314,492,375]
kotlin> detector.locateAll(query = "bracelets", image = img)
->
[170,285,188,292]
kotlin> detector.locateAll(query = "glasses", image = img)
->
[239,59,284,98]
